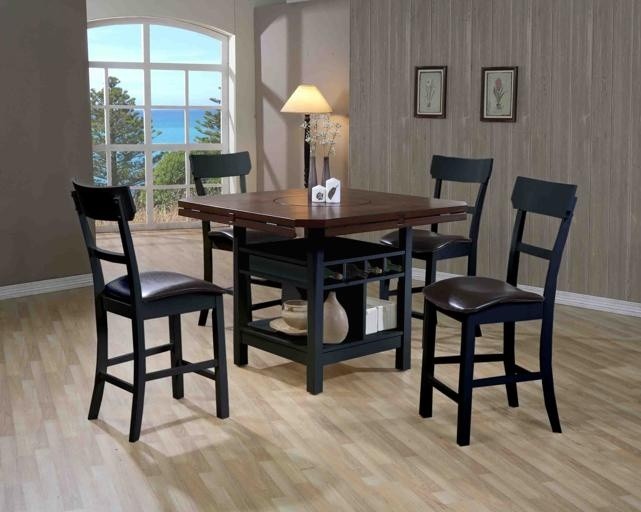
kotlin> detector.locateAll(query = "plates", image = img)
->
[269,317,308,337]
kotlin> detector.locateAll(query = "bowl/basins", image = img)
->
[281,299,308,330]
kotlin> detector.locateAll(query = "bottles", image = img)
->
[319,292,350,345]
